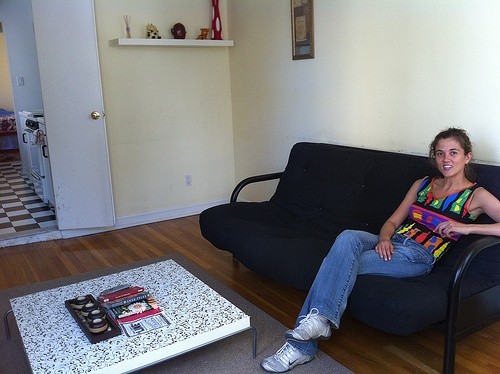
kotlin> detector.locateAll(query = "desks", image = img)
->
[3,258,258,374]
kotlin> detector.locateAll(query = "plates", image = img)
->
[65,294,122,344]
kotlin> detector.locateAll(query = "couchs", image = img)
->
[198,142,500,374]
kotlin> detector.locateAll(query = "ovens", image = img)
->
[21,127,48,205]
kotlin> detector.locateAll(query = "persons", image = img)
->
[260,127,500,373]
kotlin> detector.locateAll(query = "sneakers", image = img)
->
[261,342,314,373]
[284,307,333,343]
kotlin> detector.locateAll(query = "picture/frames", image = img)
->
[291,0,315,60]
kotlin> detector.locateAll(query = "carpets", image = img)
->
[0,250,354,374]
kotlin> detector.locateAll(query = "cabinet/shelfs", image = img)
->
[19,110,54,210]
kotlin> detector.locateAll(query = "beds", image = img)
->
[0,108,17,151]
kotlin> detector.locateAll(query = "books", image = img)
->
[97,282,161,336]
